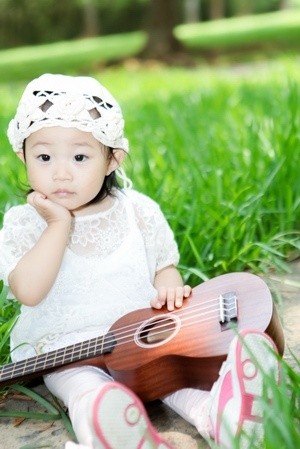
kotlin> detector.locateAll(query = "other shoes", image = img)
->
[69,384,176,448]
[209,329,282,448]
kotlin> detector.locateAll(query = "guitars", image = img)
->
[0,272,285,402]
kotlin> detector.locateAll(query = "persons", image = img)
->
[0,74,282,449]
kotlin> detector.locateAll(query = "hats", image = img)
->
[7,73,129,155]
